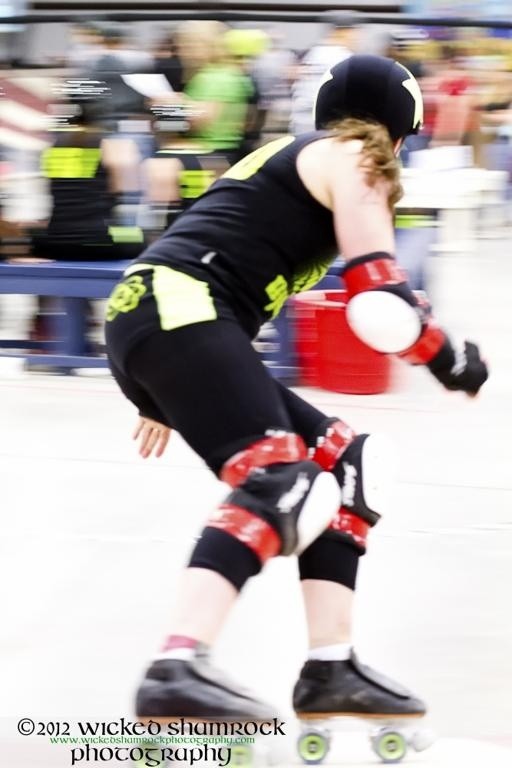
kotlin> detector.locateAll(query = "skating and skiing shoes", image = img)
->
[135,659,275,724]
[293,660,427,718]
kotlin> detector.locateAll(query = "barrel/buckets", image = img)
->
[290,288,389,394]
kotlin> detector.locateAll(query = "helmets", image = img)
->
[316,52,424,133]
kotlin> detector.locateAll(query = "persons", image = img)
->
[0,15,511,381]
[105,54,492,727]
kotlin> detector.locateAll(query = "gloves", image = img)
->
[429,332,488,394]
